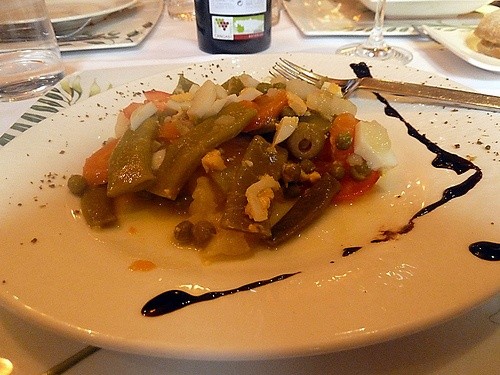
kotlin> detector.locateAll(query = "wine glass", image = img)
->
[333,0,413,68]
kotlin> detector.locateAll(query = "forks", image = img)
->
[0,18,93,37]
[269,53,500,112]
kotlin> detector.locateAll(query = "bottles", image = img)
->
[193,0,272,55]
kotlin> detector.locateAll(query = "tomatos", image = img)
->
[79,90,383,203]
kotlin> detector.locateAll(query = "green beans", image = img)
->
[81,183,118,228]
[68,175,86,193]
[107,74,372,249]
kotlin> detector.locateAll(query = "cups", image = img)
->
[0,0,65,101]
[166,0,282,25]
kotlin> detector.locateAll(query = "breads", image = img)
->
[474,7,500,59]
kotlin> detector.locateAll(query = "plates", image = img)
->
[283,0,500,36]
[0,0,165,51]
[420,21,500,72]
[0,53,500,362]
[361,0,494,18]
